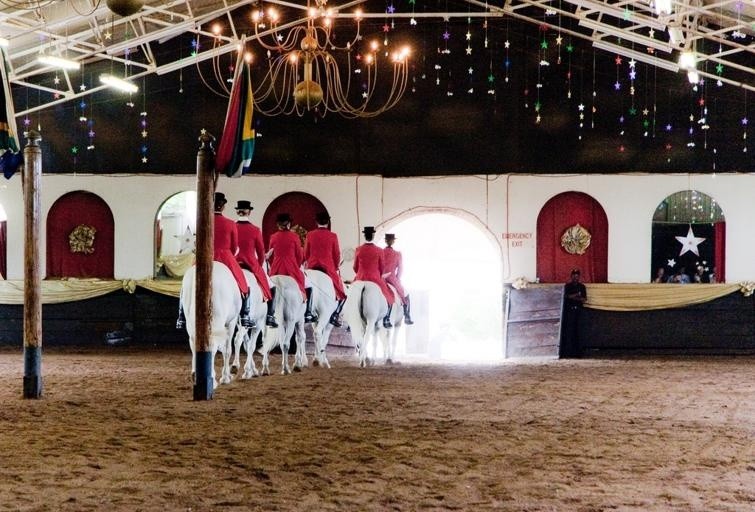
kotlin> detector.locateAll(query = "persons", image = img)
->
[235,201,278,327]
[353,226,394,330]
[305,216,347,326]
[175,192,254,328]
[652,260,714,283]
[268,214,313,324]
[564,269,587,331]
[382,234,412,324]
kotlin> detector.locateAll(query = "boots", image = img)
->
[266,287,278,327]
[383,303,392,328]
[403,305,413,324]
[241,287,255,327]
[176,288,186,330]
[330,297,346,327]
[305,287,318,323]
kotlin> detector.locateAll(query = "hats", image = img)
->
[362,227,376,233]
[385,234,396,239]
[275,214,293,222]
[235,200,253,210]
[215,193,227,203]
[315,212,330,220]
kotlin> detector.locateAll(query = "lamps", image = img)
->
[211,0,412,119]
[655,0,699,85]
[0,0,140,94]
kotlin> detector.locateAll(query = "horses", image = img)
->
[230,248,274,382]
[182,247,240,390]
[298,259,344,369]
[342,271,404,368]
[257,261,307,376]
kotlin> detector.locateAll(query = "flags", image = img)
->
[216,51,257,177]
